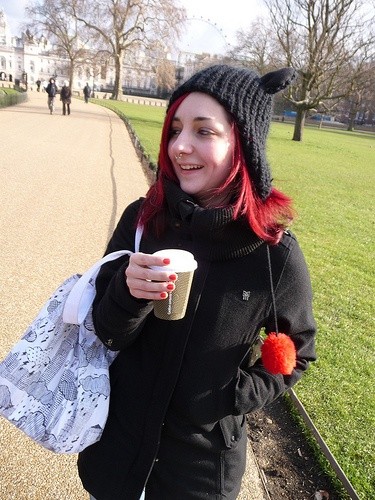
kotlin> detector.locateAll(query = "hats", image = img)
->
[167,64,296,200]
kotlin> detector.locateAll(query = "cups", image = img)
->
[147,249,198,320]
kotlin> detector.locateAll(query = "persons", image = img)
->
[83,82,91,102]
[60,82,72,115]
[44,78,57,114]
[36,79,41,92]
[76,64,318,500]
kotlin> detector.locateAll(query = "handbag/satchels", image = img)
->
[0,195,150,457]
